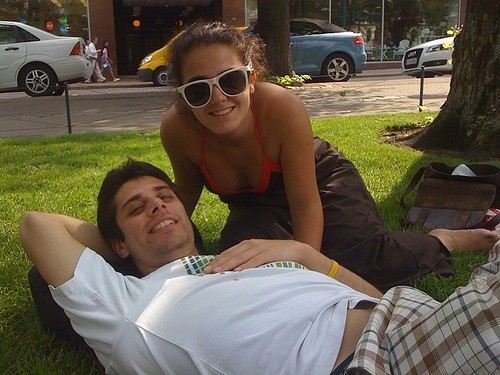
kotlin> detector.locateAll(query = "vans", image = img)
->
[136,26,246,87]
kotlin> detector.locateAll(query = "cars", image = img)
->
[402,35,455,76]
[0,20,94,96]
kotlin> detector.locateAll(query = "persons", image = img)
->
[18,157,500,375]
[160,21,500,289]
[84,36,121,83]
[397,25,430,62]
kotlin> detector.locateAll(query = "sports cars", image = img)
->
[241,18,368,82]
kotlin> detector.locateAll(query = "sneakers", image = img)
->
[96,78,99,81]
[100,78,106,83]
[113,77,121,82]
[85,79,91,83]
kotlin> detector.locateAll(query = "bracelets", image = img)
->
[326,259,340,281]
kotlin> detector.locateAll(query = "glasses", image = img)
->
[176,61,254,109]
